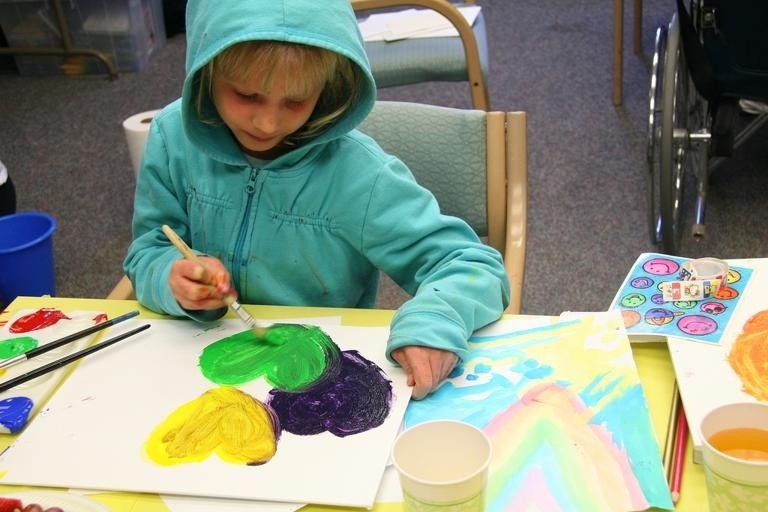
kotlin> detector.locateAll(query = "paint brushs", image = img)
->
[0,323,151,394]
[159,221,268,341]
[0,309,141,371]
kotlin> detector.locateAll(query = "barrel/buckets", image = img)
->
[0,212,58,302]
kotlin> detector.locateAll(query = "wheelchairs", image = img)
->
[645,0,767,255]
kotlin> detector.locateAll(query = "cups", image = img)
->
[700,400,768,511]
[391,420,493,512]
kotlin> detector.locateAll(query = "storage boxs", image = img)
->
[0,0,168,76]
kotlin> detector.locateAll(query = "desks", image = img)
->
[0,296,709,512]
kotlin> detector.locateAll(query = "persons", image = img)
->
[122,0,509,400]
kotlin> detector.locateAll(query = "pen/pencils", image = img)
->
[662,375,687,505]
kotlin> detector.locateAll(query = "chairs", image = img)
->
[348,1,492,110]
[105,0,526,315]
